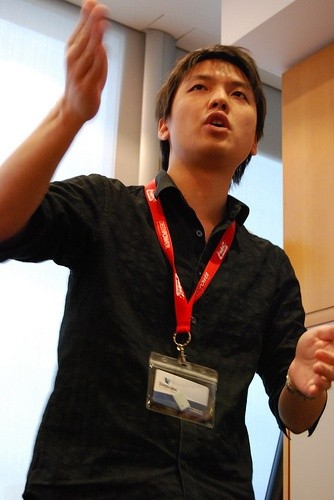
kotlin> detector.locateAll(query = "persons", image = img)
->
[0,1,334,500]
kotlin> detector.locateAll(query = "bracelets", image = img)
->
[284,374,317,402]
